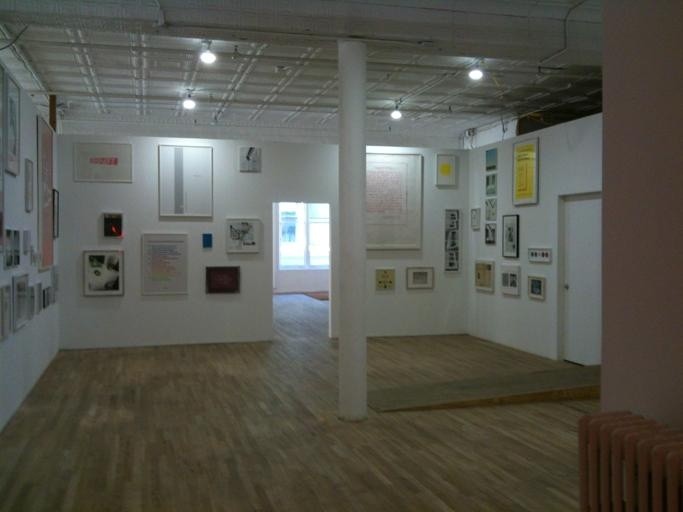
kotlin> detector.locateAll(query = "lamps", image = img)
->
[468,54,487,81]
[391,97,404,119]
[200,34,216,66]
[182,88,196,109]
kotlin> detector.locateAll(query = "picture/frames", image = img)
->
[363,151,424,251]
[224,218,261,253]
[404,266,434,290]
[140,232,188,296]
[1,61,136,346]
[433,135,559,304]
[157,145,213,218]
[205,265,241,293]
[375,267,396,293]
[237,145,263,173]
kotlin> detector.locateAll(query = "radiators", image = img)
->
[569,406,682,512]
